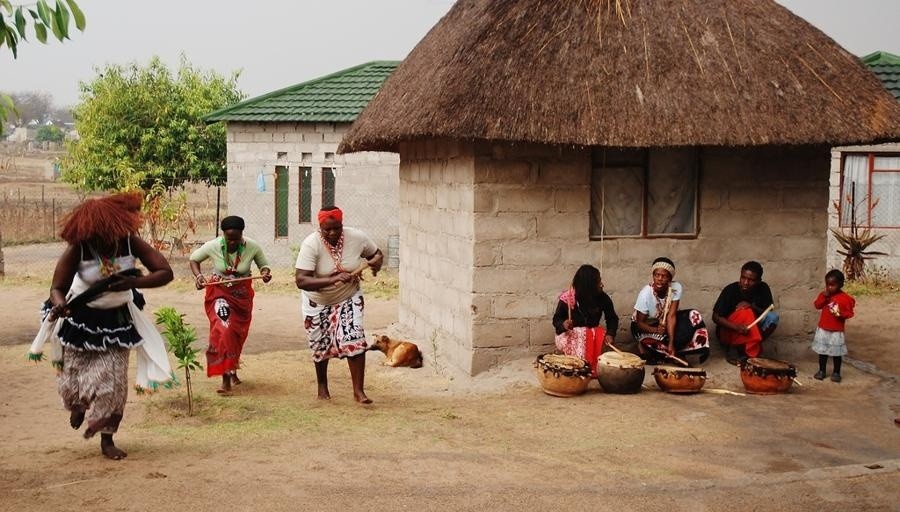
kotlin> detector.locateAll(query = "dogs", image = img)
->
[369,334,423,369]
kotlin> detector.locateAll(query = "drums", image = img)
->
[738,358,801,395]
[597,351,646,394]
[534,353,593,396]
[651,366,710,393]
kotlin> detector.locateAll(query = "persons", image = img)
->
[630,255,710,368]
[293,206,383,404]
[552,262,620,379]
[712,260,780,366]
[813,270,856,383]
[25,190,181,460]
[188,215,273,395]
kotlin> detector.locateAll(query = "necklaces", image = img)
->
[652,286,669,325]
[88,240,121,282]
[317,229,345,277]
[220,237,248,280]
